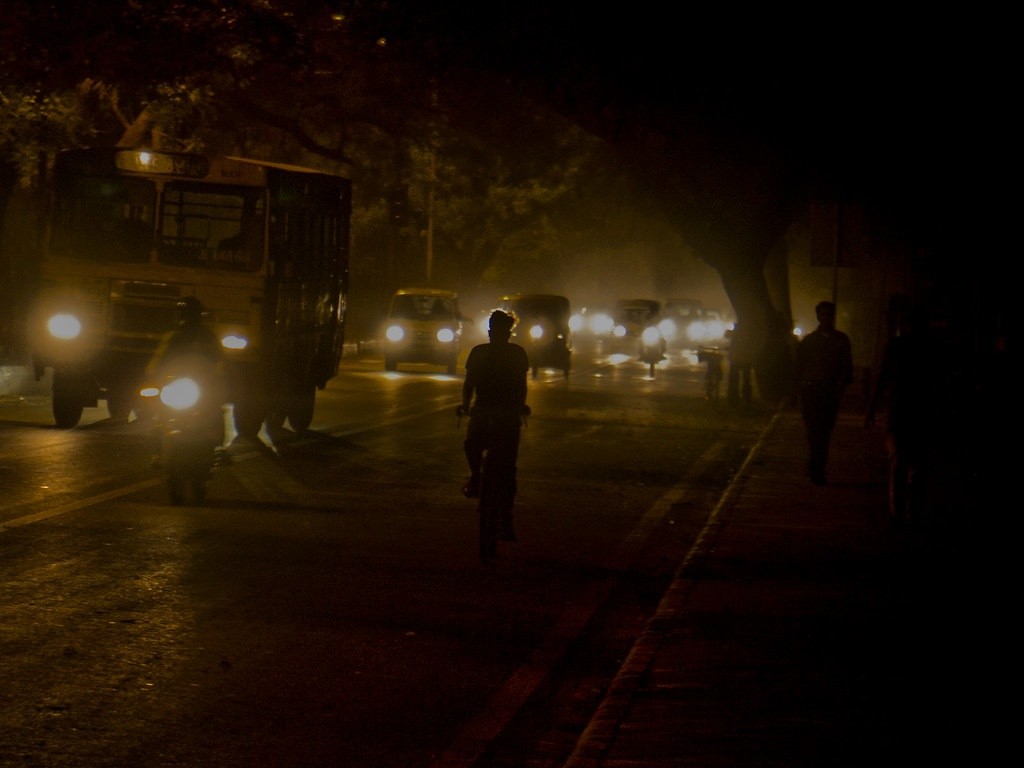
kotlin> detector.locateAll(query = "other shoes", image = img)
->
[463,473,479,494]
[808,466,827,485]
[497,514,512,539]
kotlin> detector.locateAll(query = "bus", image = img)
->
[34,146,352,437]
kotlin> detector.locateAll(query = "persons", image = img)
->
[142,297,227,451]
[456,310,532,541]
[863,303,931,543]
[790,301,854,487]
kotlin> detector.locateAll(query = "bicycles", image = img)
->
[697,346,725,407]
[458,406,536,564]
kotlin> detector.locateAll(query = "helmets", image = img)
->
[176,296,202,324]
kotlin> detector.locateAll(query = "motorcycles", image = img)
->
[492,294,573,378]
[140,373,212,503]
[639,340,667,377]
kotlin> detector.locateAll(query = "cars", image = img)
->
[605,299,660,342]
[383,288,470,374]
[663,299,712,345]
[573,304,611,340]
